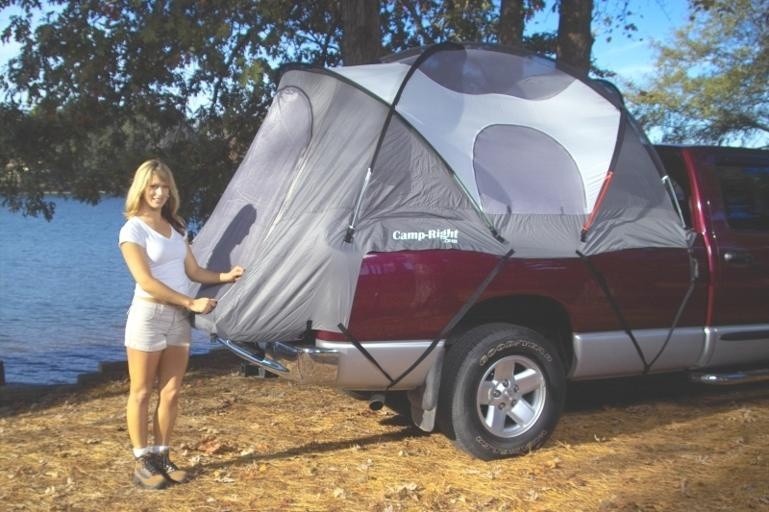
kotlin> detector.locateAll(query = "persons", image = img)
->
[118,159,245,489]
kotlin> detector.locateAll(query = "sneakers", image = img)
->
[134,454,167,489]
[151,449,190,483]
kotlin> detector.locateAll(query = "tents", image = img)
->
[186,39,691,343]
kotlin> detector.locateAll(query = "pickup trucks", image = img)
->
[213,146,769,462]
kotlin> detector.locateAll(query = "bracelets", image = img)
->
[216,271,223,282]
